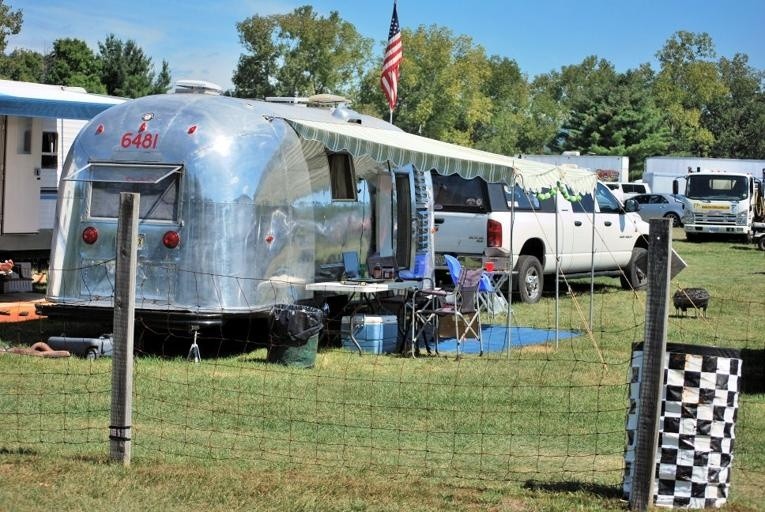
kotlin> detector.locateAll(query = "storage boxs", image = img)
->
[438,314,478,340]
[341,315,398,352]
[481,256,510,270]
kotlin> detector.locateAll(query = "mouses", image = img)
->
[395,279,403,282]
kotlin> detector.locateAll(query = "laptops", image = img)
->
[342,252,385,283]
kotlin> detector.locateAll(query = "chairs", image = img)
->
[444,253,498,324]
[411,266,484,361]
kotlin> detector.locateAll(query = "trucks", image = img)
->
[682,171,763,241]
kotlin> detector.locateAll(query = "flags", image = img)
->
[378,0,405,111]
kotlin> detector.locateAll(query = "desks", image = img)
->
[483,270,518,310]
[305,280,418,356]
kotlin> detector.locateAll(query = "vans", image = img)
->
[431,169,651,304]
[603,182,651,206]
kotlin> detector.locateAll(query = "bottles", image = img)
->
[382,265,394,280]
[373,263,382,279]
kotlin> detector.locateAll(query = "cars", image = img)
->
[624,193,689,227]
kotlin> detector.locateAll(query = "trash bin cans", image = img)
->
[269,304,323,368]
[621,340,745,510]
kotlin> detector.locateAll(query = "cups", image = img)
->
[485,261,495,272]
